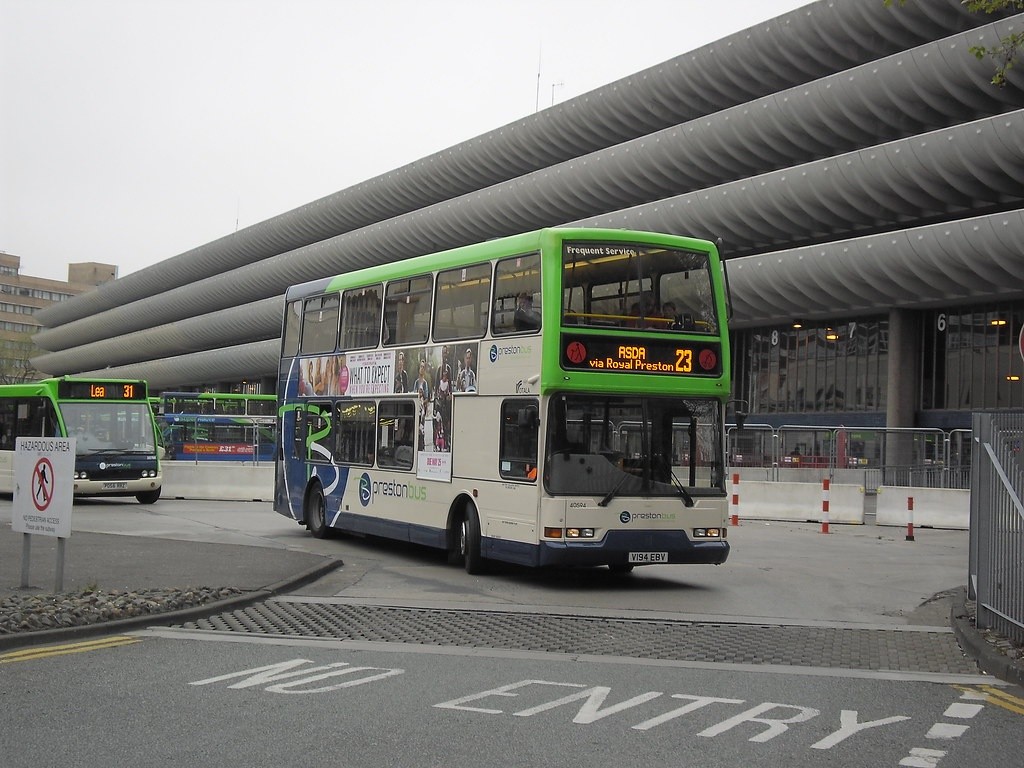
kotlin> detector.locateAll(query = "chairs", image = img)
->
[562,309,578,325]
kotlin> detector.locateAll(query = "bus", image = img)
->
[85,391,277,461]
[38,374,167,504]
[0,382,67,495]
[273,226,752,574]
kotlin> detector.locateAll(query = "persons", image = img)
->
[394,345,475,452]
[307,354,346,396]
[790,447,801,467]
[626,301,680,330]
[514,291,541,332]
